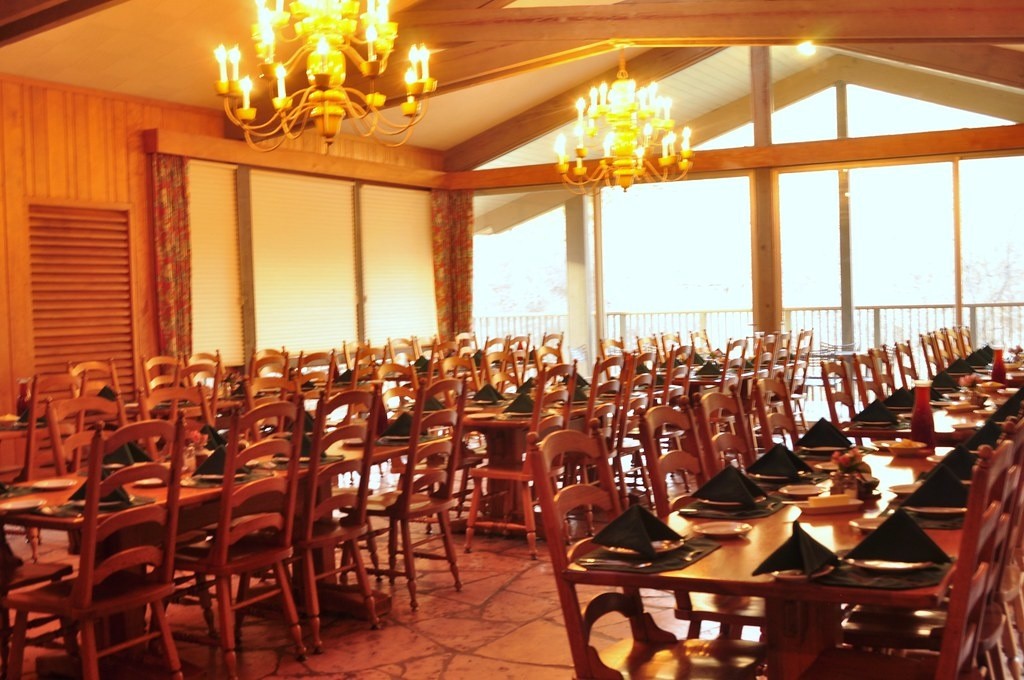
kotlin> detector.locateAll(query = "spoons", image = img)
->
[582,559,651,571]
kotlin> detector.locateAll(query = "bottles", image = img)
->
[992,349,1006,385]
[912,379,933,447]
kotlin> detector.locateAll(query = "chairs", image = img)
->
[0,325,1024,680]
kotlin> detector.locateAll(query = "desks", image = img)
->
[564,442,956,680]
[0,433,454,680]
[672,359,806,452]
[409,385,684,541]
[842,387,1021,446]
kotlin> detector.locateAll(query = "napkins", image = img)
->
[191,446,251,475]
[383,411,427,435]
[63,466,134,505]
[105,440,154,465]
[965,420,1012,449]
[922,446,976,479]
[502,392,544,414]
[591,500,681,554]
[745,443,813,481]
[798,415,851,445]
[749,516,839,580]
[851,398,897,427]
[692,463,771,516]
[472,382,504,403]
[906,466,971,508]
[271,431,327,458]
[514,377,539,392]
[199,424,225,449]
[287,411,316,429]
[845,507,950,567]
[883,387,915,406]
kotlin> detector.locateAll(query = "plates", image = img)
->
[845,555,935,571]
[816,462,841,471]
[928,456,946,462]
[746,472,811,481]
[870,440,927,448]
[947,346,1024,383]
[412,379,669,428]
[780,484,822,497]
[696,496,766,508]
[888,484,922,495]
[601,539,684,556]
[853,387,1024,440]
[771,565,834,576]
[798,444,845,450]
[693,521,753,540]
[0,435,433,509]
[650,349,800,378]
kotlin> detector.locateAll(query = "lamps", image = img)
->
[213,0,438,154]
[554,53,696,197]
